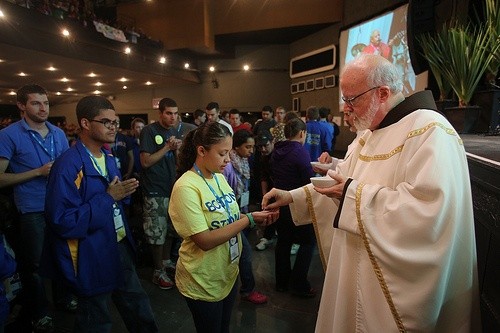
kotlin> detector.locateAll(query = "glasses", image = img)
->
[92,119,120,129]
[342,87,379,104]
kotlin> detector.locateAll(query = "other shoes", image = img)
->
[61,294,77,313]
[256,238,273,250]
[291,243,299,254]
[242,292,267,304]
[276,288,289,292]
[293,287,317,297]
[164,261,175,275]
[31,312,55,333]
[152,270,174,289]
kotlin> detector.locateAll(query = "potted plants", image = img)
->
[415,0,500,134]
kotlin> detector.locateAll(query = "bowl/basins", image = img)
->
[311,161,333,170]
[310,176,339,189]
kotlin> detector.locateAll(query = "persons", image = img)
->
[362,29,405,65]
[0,82,162,333]
[262,53,482,333]
[138,97,341,333]
[36,0,136,39]
[318,101,362,177]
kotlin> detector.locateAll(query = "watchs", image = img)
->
[246,213,256,228]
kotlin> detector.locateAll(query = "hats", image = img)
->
[255,131,272,146]
